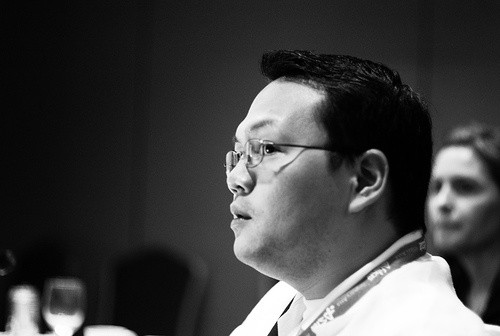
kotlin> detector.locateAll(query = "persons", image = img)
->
[426,123,500,326]
[224,49,500,336]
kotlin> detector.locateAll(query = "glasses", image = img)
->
[224,139,349,173]
